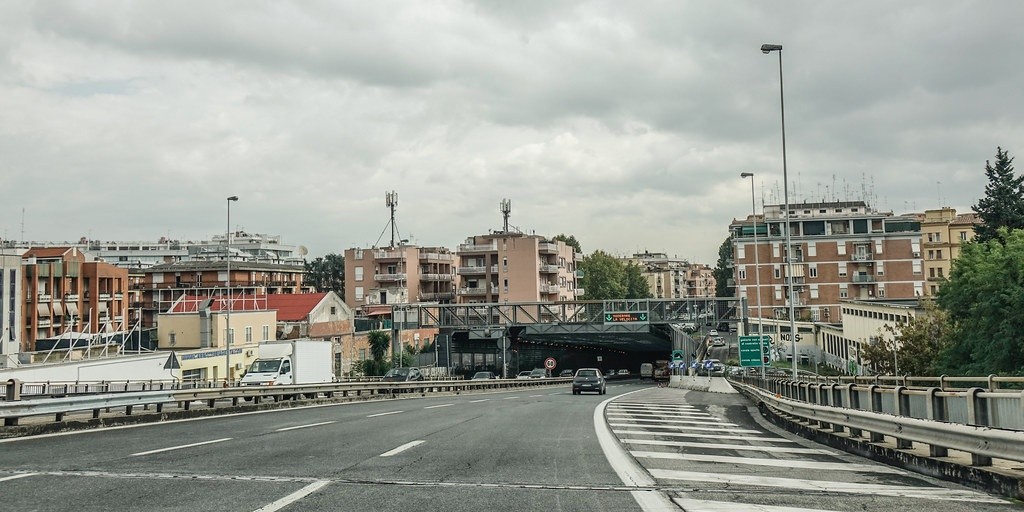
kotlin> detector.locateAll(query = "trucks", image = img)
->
[240,338,335,398]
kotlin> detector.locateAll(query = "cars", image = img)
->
[377,309,736,393]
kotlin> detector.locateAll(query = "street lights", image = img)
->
[226,194,240,390]
[739,42,801,379]
